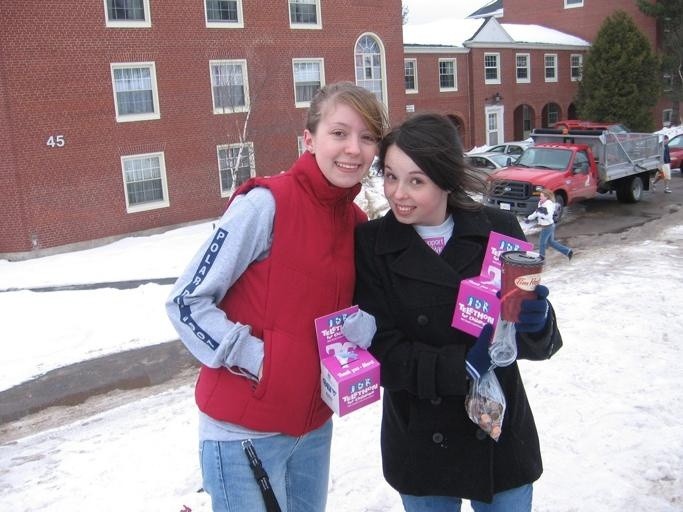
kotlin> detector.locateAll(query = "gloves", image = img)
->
[465,323,520,383]
[496,284,550,335]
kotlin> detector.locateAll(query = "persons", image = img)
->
[523,189,574,261]
[164,76,390,510]
[350,111,565,511]
[651,134,674,194]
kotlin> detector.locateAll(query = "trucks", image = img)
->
[482,130,664,224]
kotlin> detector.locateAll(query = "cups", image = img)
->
[499,250,546,323]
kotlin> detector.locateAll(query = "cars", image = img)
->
[632,125,683,176]
[463,137,535,180]
